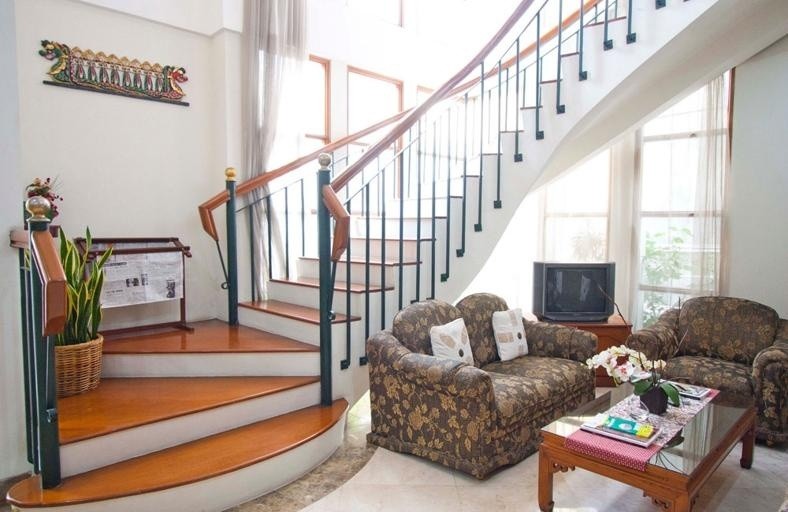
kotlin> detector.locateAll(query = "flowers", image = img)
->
[584,345,683,406]
[25,178,64,213]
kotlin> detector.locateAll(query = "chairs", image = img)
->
[626,296,788,446]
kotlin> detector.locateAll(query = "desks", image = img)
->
[546,313,632,386]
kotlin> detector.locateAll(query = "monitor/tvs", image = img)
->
[533,262,615,323]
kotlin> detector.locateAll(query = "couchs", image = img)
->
[361,293,599,481]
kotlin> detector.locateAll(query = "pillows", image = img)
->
[490,308,529,361]
[427,318,474,370]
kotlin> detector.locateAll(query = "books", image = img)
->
[603,415,656,440]
[664,380,712,400]
[577,412,662,448]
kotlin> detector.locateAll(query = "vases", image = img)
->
[23,201,58,232]
[641,386,670,415]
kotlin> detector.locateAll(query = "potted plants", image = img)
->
[25,225,117,400]
